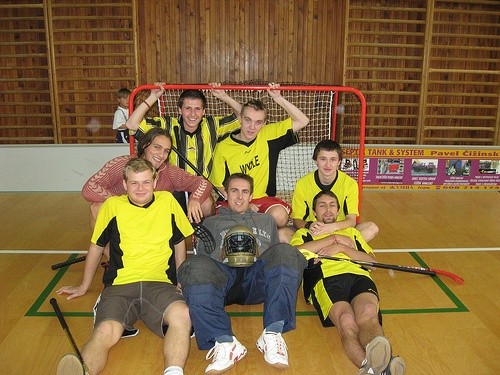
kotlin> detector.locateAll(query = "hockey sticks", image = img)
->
[49,295,92,375]
[51,257,89,269]
[318,254,466,285]
[170,145,227,202]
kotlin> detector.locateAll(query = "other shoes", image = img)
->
[56,353,84,375]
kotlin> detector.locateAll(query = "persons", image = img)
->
[53,159,196,375]
[177,173,308,375]
[82,127,212,341]
[113,88,137,143]
[289,189,406,375]
[198,81,310,229]
[279,140,379,246]
[126,80,244,220]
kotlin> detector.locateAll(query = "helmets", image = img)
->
[221,226,259,267]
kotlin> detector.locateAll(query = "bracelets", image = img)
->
[142,99,151,110]
[330,233,338,244]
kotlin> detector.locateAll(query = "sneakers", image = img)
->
[378,356,406,375]
[256,328,288,369]
[204,336,247,375]
[358,336,392,375]
